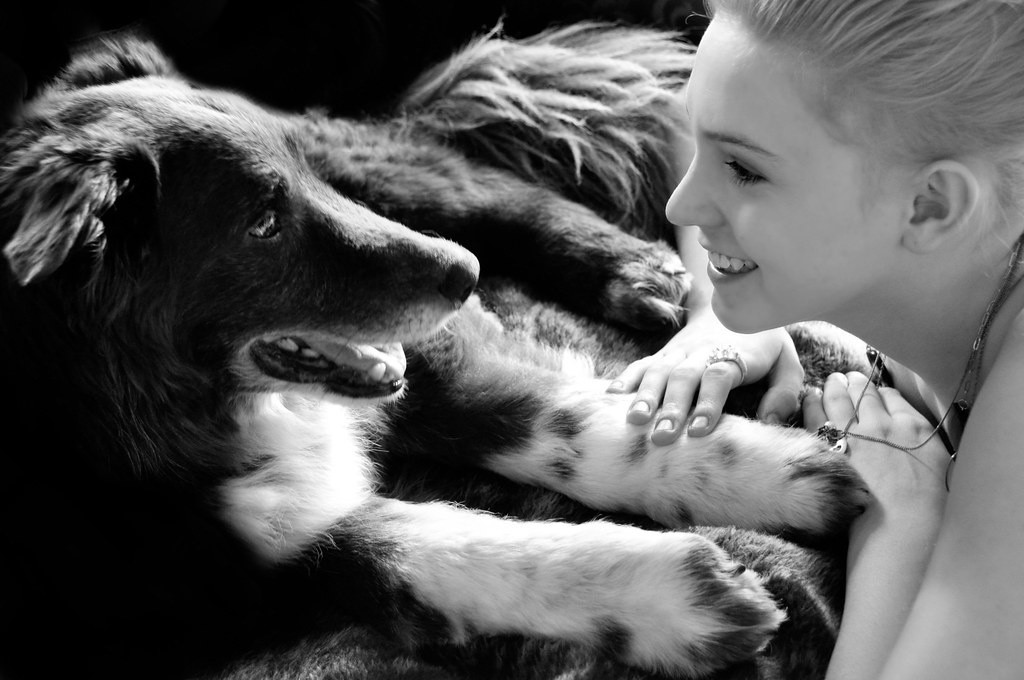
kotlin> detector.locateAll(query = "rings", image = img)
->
[705,343,748,390]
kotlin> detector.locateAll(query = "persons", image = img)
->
[605,0,1024,680]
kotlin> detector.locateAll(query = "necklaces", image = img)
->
[816,235,1024,454]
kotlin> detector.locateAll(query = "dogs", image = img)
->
[1,19,871,680]
